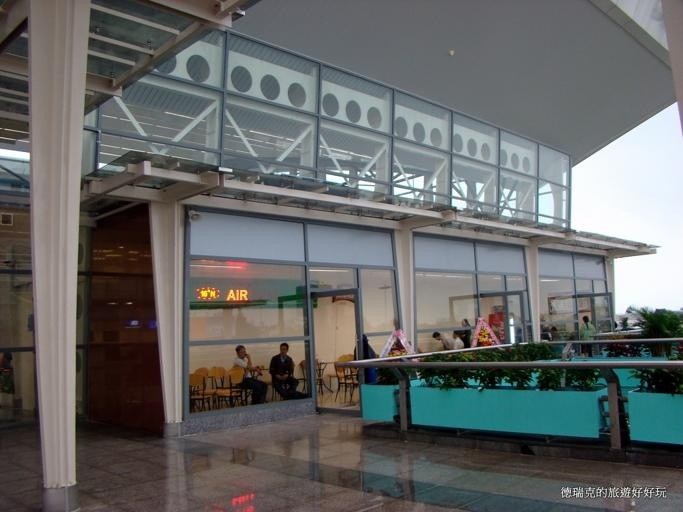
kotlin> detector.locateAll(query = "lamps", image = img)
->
[189,352,360,413]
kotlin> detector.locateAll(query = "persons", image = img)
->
[516,322,566,343]
[453,331,464,348]
[268,342,301,400]
[431,330,454,354]
[0,351,12,371]
[233,344,267,406]
[579,315,595,358]
[461,317,472,349]
[351,334,376,383]
